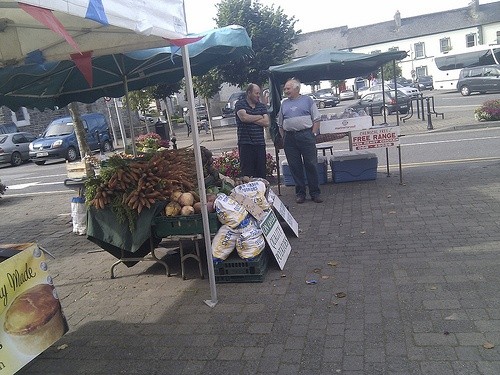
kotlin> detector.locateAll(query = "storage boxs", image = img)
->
[281,155,328,186]
[155,210,220,236]
[329,151,378,183]
[213,242,272,283]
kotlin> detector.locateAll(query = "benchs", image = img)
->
[166,233,215,279]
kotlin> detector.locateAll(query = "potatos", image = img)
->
[235,175,253,187]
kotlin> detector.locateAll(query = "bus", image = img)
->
[432,44,500,90]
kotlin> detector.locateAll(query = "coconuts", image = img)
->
[165,191,217,216]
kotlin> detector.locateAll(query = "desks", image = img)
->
[84,199,172,279]
[275,127,404,195]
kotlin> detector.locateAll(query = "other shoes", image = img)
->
[311,195,322,202]
[296,195,305,202]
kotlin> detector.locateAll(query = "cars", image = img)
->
[361,83,420,99]
[356,77,433,98]
[0,132,37,167]
[344,89,409,116]
[340,90,355,100]
[140,92,243,121]
[0,121,18,133]
[457,64,500,96]
[305,93,340,108]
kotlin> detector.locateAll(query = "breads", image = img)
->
[3,284,60,334]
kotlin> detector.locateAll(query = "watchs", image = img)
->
[312,132,316,136]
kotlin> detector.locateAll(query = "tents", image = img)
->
[0,0,406,307]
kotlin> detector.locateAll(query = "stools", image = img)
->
[316,144,333,156]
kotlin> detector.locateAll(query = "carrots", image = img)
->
[93,146,200,214]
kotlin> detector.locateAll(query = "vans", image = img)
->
[28,112,112,165]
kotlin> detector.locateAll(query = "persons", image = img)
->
[155,117,162,125]
[186,110,212,134]
[277,79,324,203]
[235,83,269,180]
